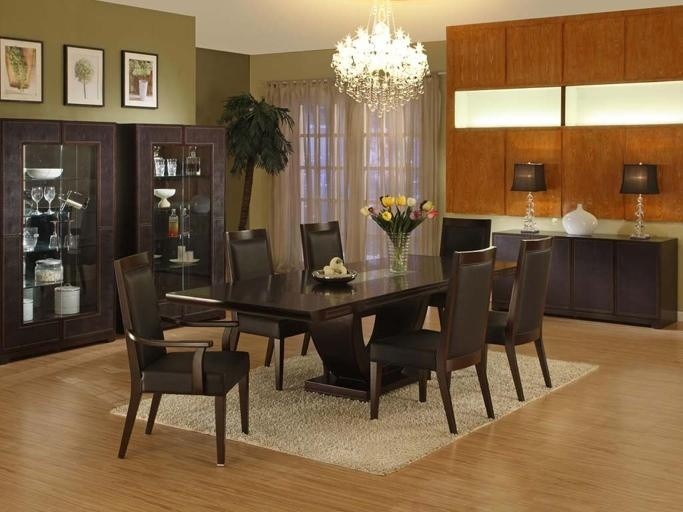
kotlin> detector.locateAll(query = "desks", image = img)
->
[165,253,516,403]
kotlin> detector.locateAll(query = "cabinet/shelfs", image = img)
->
[492,233,572,321]
[119,123,227,328]
[570,238,677,330]
[2,120,117,359]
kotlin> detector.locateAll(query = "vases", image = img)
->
[562,203,598,236]
[384,229,412,278]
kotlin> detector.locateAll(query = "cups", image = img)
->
[153,158,178,177]
[176,245,194,262]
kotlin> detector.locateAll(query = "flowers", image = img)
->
[360,193,439,270]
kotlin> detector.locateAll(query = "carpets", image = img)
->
[110,341,598,475]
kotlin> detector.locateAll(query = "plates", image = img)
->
[167,258,200,265]
[311,269,358,283]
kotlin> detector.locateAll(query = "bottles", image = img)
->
[168,207,190,239]
[184,152,203,176]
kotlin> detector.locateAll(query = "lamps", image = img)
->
[511,162,547,233]
[620,163,661,241]
[328,1,430,118]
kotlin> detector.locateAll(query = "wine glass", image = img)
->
[30,186,55,216]
[48,219,75,251]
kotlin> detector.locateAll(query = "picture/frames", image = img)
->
[61,45,104,108]
[120,50,158,110]
[0,36,44,104]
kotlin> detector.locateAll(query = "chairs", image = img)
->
[365,244,494,436]
[112,249,250,468]
[224,227,279,366]
[297,220,343,355]
[482,236,552,402]
[426,217,493,326]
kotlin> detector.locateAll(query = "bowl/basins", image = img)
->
[21,227,40,251]
[26,167,63,182]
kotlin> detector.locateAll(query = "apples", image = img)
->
[323,256,348,276]
